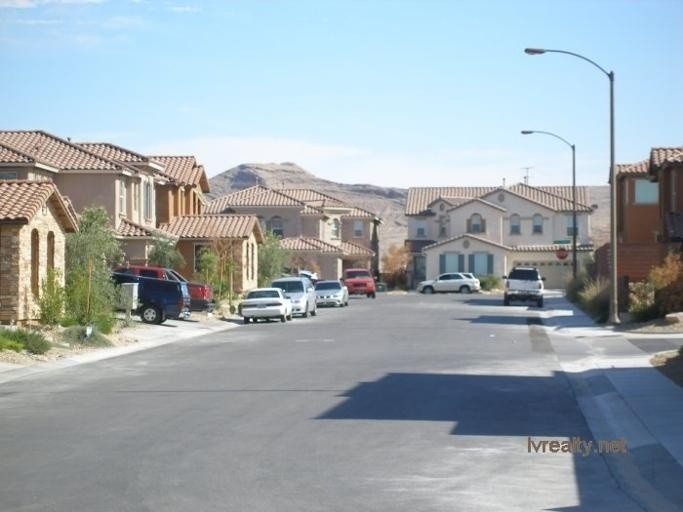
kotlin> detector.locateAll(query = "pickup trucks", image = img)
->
[109,271,190,324]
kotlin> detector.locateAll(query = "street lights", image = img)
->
[525,48,621,324]
[521,130,576,281]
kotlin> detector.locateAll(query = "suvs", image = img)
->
[503,264,546,307]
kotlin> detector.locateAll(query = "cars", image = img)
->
[169,270,211,314]
[270,277,316,317]
[314,281,348,308]
[415,273,480,294]
[339,269,376,299]
[241,288,292,323]
